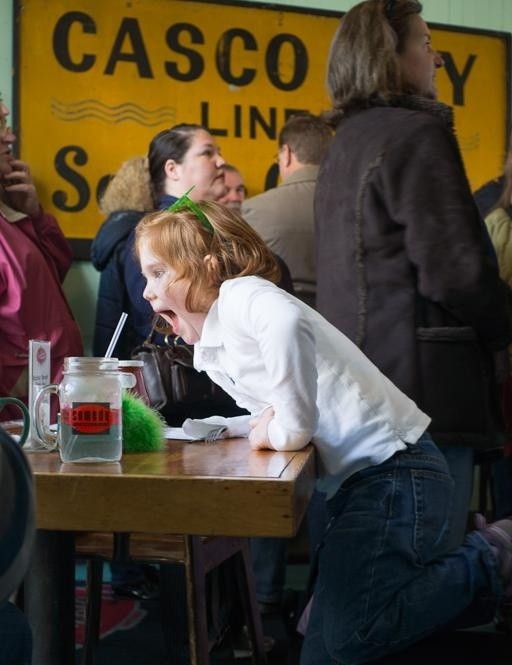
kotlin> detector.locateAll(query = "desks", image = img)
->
[24,428,315,664]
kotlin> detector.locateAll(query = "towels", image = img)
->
[165,414,250,442]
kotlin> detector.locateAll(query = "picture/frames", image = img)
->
[13,1,512,264]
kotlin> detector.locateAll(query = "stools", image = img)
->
[75,531,264,664]
[388,606,512,665]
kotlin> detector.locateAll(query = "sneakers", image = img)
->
[232,625,274,658]
[484,518,512,579]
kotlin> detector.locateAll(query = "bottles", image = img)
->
[118,358,150,402]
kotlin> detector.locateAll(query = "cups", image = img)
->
[36,357,124,465]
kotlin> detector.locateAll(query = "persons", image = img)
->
[90,123,294,429]
[485,163,512,284]
[240,116,333,305]
[133,187,512,665]
[0,117,86,665]
[313,1,499,408]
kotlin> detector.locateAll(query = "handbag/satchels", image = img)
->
[130,343,222,411]
